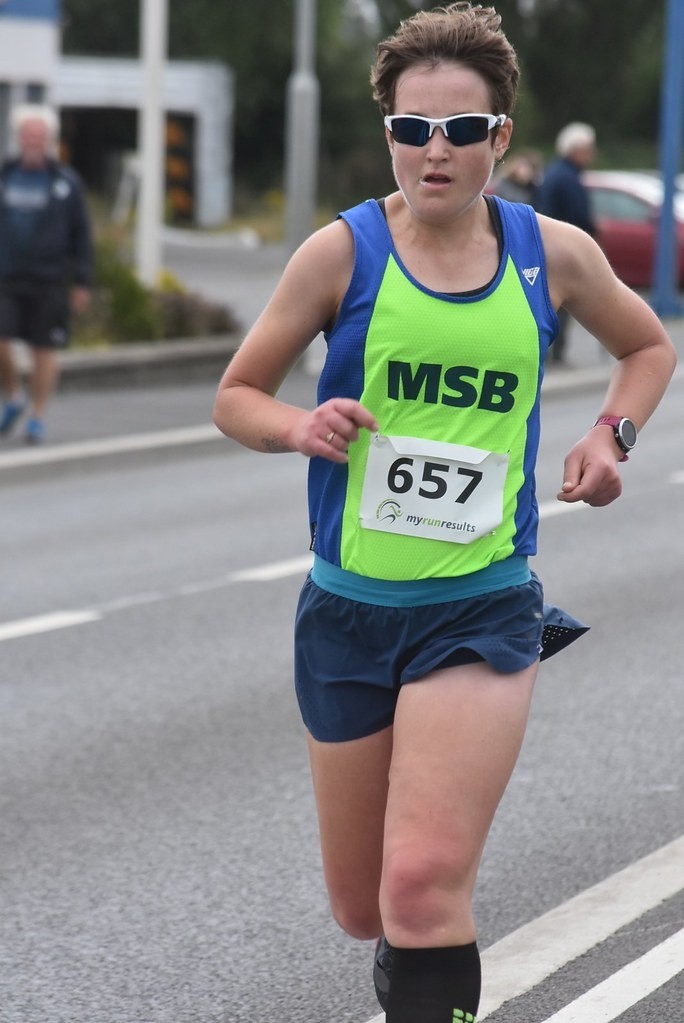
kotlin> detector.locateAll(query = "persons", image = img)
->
[215,1,680,1023]
[0,104,96,445]
[493,121,596,370]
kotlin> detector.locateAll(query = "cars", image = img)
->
[483,166,684,295]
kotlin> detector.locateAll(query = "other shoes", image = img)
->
[0,398,28,433]
[27,419,42,439]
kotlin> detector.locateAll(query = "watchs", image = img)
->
[593,416,638,462]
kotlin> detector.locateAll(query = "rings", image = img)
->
[326,431,335,443]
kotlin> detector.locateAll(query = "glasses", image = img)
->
[383,112,507,147]
